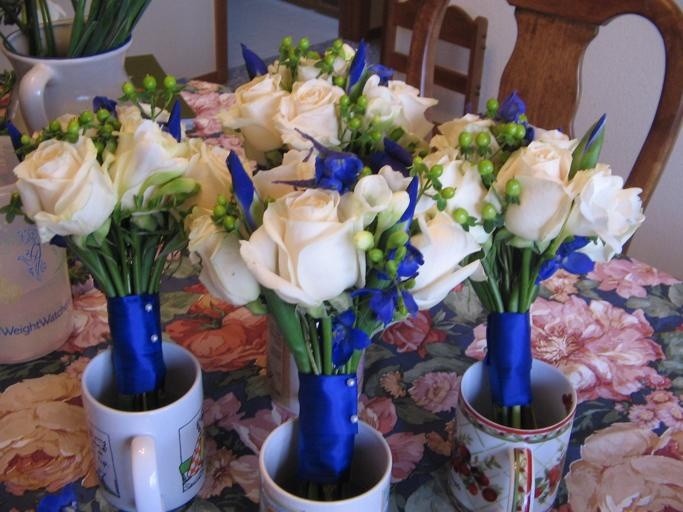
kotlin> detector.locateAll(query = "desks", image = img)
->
[213,0,381,85]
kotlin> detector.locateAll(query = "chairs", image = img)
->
[382,0,683,210]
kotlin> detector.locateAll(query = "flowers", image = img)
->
[14,38,644,335]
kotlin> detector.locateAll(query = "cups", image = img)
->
[0,181,75,366]
